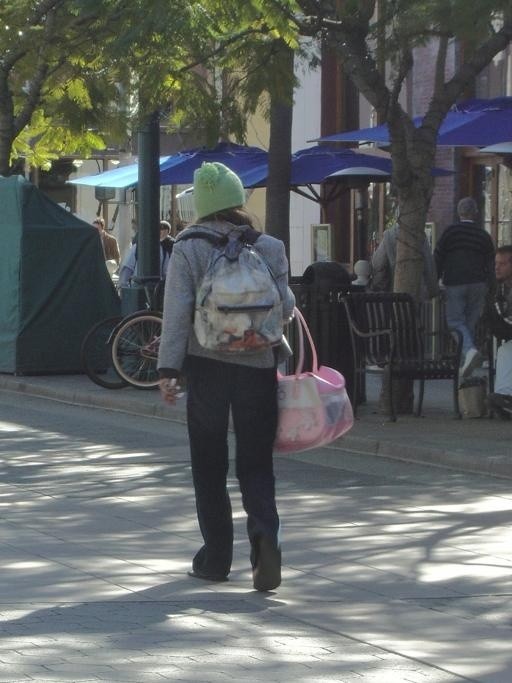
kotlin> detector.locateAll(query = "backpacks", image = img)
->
[177,227,283,351]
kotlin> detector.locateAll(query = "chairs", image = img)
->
[340,291,464,421]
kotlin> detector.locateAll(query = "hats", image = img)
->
[193,163,245,220]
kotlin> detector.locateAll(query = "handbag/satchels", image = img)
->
[273,366,354,453]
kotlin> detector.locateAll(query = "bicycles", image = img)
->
[107,309,173,389]
[81,276,164,388]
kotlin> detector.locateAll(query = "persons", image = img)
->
[432,194,493,376]
[491,245,512,340]
[372,209,438,297]
[88,215,177,294]
[154,160,303,592]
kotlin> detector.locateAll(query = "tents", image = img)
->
[0,174,137,377]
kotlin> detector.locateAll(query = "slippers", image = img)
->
[254,550,281,591]
[188,570,228,582]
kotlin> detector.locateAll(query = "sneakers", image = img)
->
[463,349,479,377]
[484,392,512,415]
